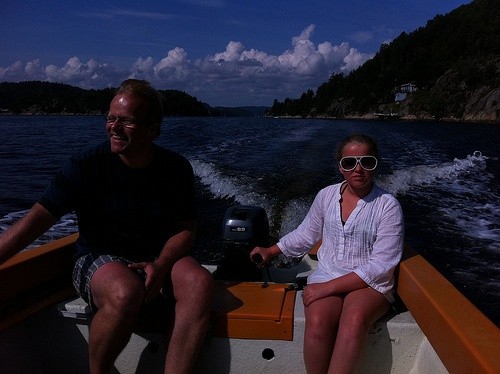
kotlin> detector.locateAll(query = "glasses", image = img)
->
[104,118,151,129]
[340,155,378,173]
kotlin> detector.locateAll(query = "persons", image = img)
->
[250,134,404,374]
[0,79,214,374]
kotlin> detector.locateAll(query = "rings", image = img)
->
[159,288,164,294]
